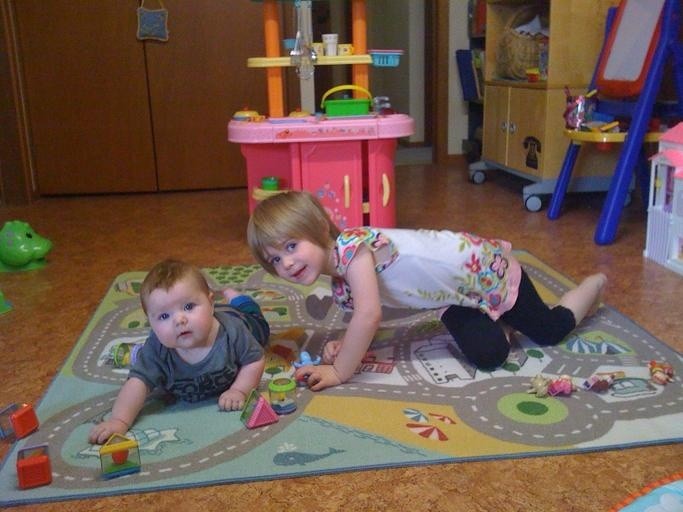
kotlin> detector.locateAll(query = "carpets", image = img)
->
[0,249,682,507]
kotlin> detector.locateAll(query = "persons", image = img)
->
[87,255,270,444]
[246,188,608,393]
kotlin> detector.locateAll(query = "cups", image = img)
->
[312,33,354,57]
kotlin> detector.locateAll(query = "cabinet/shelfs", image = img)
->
[5,0,285,197]
[465,0,637,212]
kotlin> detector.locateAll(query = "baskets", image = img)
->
[494,3,547,81]
